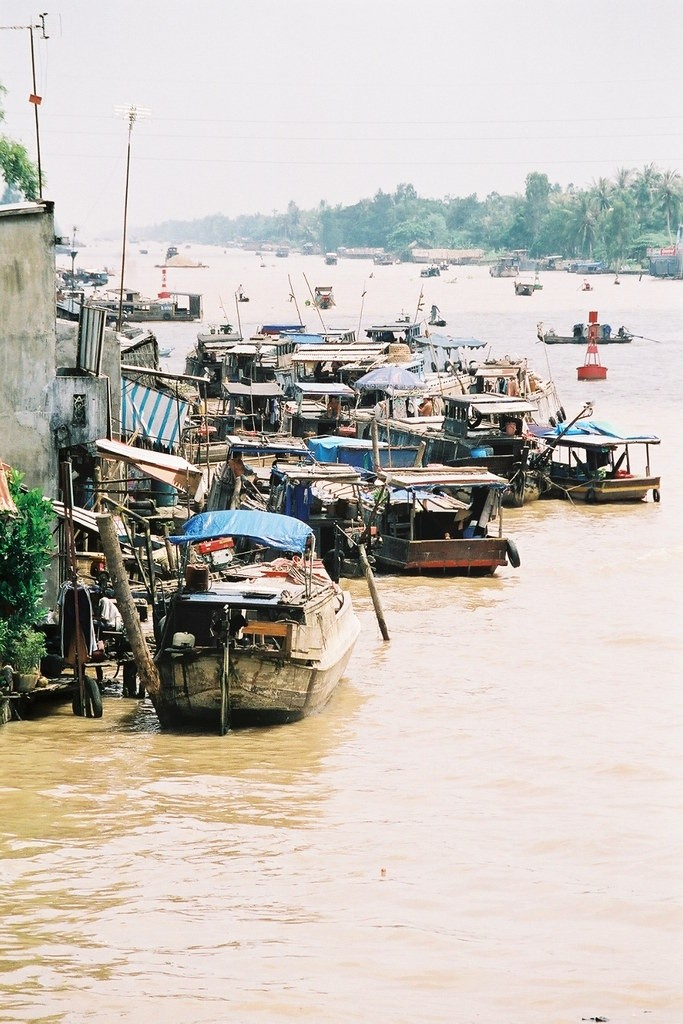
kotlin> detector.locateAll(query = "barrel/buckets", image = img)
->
[463,527,476,538]
[151,478,178,506]
[186,565,209,591]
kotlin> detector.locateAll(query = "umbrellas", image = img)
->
[354,366,427,418]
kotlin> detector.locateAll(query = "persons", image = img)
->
[236,284,245,300]
[269,453,289,486]
[324,397,343,420]
[410,395,433,416]
[228,451,259,488]
[313,361,343,378]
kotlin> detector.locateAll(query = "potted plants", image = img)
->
[373,488,389,507]
[12,625,47,690]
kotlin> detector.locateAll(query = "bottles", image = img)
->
[83,476,94,509]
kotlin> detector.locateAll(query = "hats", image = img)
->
[454,509,472,521]
[422,394,430,399]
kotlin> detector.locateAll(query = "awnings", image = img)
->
[19,488,132,541]
[94,438,206,507]
[121,364,210,385]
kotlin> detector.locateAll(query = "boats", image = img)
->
[55,245,665,736]
